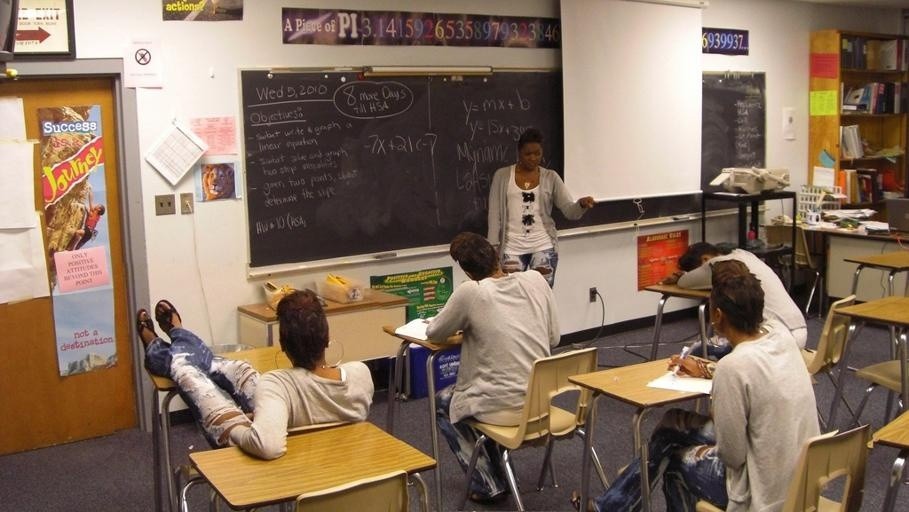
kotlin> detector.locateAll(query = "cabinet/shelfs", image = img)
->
[806,26,908,205]
[699,192,797,308]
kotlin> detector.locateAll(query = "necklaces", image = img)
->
[517,164,539,189]
[316,364,329,370]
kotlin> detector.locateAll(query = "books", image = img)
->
[395,317,463,340]
[837,37,901,206]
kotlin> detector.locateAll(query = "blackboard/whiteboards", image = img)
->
[237,64,766,279]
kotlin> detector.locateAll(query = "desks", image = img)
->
[828,293,909,430]
[770,217,909,305]
[841,241,909,301]
[227,284,413,400]
[643,283,711,362]
[383,320,567,491]
[145,343,438,510]
[867,407,909,512]
[566,354,719,510]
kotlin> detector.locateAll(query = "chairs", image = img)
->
[849,353,906,429]
[762,222,826,321]
[693,421,873,511]
[805,293,861,434]
[456,345,601,510]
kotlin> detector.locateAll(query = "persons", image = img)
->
[135,289,375,460]
[673,242,809,364]
[426,230,561,504]
[66,187,106,250]
[488,128,597,285]
[568,260,823,511]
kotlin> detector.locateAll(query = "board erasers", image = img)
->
[673,217,689,220]
[373,253,396,259]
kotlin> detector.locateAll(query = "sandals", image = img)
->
[569,489,599,511]
[155,299,182,336]
[469,492,509,506]
[137,309,158,350]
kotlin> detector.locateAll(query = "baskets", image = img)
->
[796,184,842,222]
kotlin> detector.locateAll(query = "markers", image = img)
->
[671,346,691,377]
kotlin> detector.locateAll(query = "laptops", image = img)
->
[884,197,908,232]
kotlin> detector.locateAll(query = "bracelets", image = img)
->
[695,359,709,379]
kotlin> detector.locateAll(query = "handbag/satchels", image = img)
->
[262,280,285,313]
